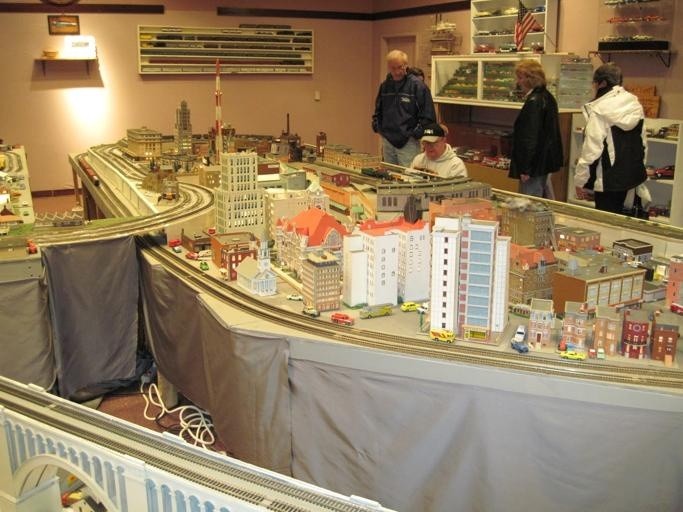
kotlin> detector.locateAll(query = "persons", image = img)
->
[371,49,437,169]
[574,61,647,213]
[507,58,563,201]
[406,67,424,83]
[410,125,468,179]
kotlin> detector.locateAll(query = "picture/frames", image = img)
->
[47,14,80,36]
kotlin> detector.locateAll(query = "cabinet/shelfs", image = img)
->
[588,1,672,70]
[136,24,314,78]
[433,101,568,202]
[424,25,456,80]
[565,112,682,228]
[429,51,569,106]
[470,1,557,53]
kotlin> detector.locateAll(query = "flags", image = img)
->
[514,0,544,52]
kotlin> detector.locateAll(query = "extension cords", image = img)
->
[141,366,157,383]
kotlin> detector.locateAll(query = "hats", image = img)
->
[421,123,444,143]
